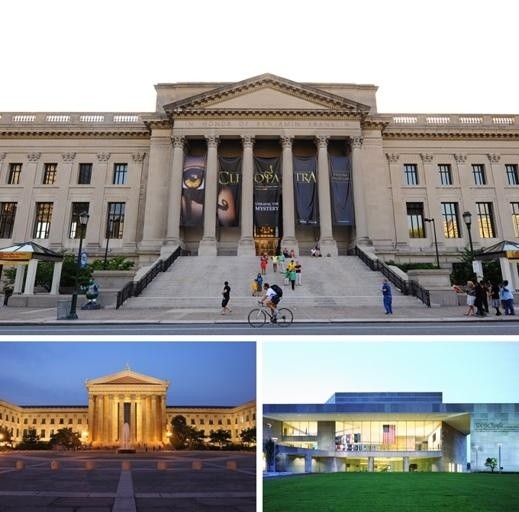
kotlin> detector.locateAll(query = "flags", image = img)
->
[382,424,397,445]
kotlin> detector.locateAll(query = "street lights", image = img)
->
[62,208,92,320]
[99,219,117,271]
[497,441,503,474]
[461,209,478,283]
[271,437,278,472]
[423,217,440,269]
[471,445,480,472]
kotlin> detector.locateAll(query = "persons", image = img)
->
[2,282,15,308]
[257,281,281,323]
[460,278,515,317]
[310,246,317,257]
[376,277,393,315]
[249,244,304,297]
[179,154,242,232]
[220,280,232,316]
[314,244,322,257]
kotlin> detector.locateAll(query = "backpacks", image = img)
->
[271,285,282,297]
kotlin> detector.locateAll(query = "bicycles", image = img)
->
[245,299,296,327]
